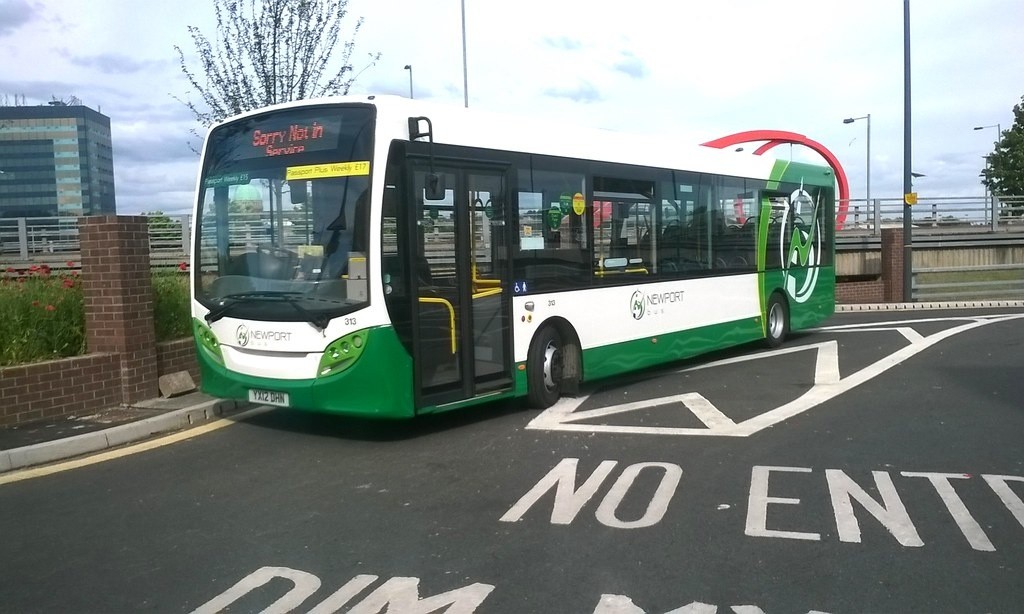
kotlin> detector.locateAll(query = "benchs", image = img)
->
[522,249,591,275]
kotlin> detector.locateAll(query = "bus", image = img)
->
[189,93,837,419]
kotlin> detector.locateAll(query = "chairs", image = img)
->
[640,216,810,273]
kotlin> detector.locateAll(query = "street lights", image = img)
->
[404,65,413,99]
[843,113,870,228]
[973,124,1004,218]
